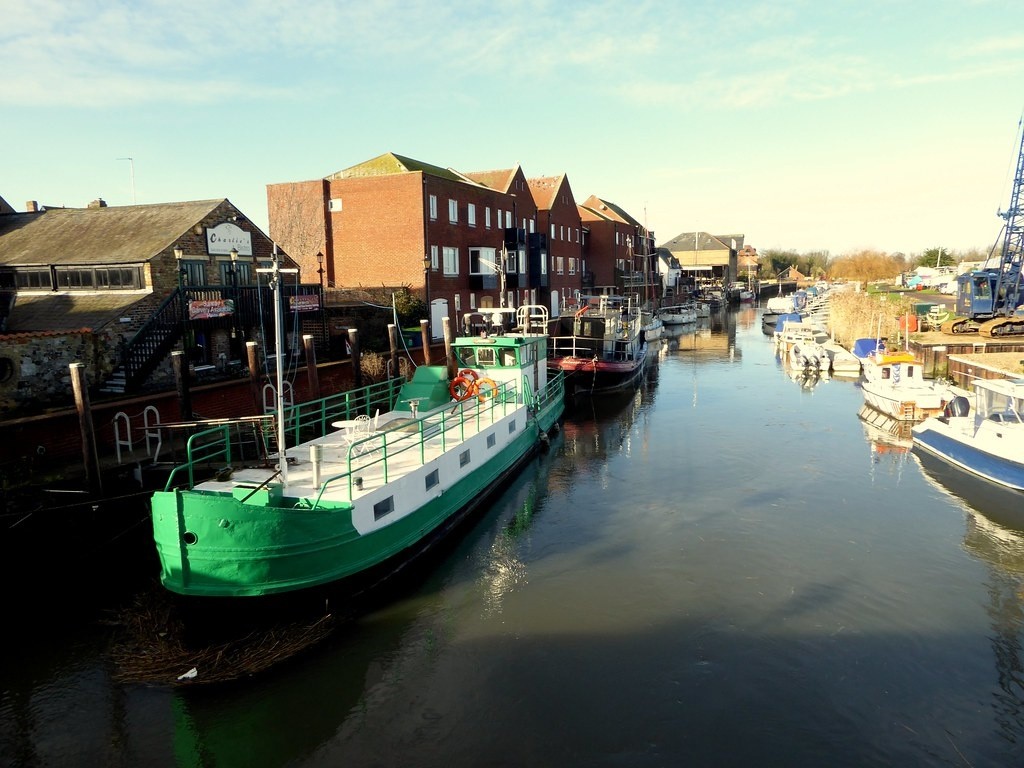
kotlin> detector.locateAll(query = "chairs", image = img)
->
[351,415,370,450]
[358,409,380,457]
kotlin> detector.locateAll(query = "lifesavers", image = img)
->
[458,368,478,392]
[473,377,498,402]
[449,376,473,401]
[575,305,589,318]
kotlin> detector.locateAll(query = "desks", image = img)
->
[194,365,216,381]
[331,420,363,462]
[266,353,286,368]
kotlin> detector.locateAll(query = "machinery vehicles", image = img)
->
[940,119,1024,339]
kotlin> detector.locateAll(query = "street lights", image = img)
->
[230,248,245,369]
[172,243,188,364]
[421,254,432,344]
[317,252,327,360]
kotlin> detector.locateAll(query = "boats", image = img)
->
[861,312,948,421]
[762,276,831,371]
[640,300,711,341]
[547,296,647,422]
[149,242,565,652]
[911,378,1024,490]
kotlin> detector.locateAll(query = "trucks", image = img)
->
[939,281,958,295]
